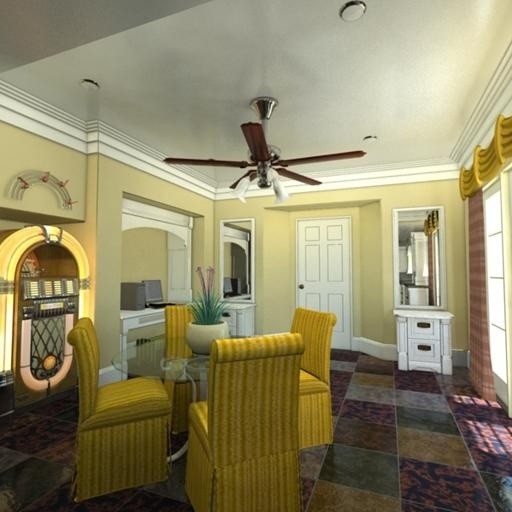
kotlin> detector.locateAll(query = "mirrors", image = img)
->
[390,205,448,311]
[217,217,256,303]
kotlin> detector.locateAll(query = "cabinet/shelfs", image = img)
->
[204,304,255,337]
[391,307,455,376]
[399,271,429,306]
[409,232,429,286]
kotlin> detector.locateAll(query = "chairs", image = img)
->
[67,317,173,501]
[163,305,201,433]
[291,306,337,449]
[181,331,303,512]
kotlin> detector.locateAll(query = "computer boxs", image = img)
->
[120,282,145,310]
[231,278,242,295]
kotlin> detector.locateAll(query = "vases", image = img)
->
[186,320,229,355]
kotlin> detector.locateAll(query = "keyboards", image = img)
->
[149,303,175,307]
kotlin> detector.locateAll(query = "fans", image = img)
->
[160,96,368,205]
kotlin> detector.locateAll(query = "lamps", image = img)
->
[230,161,292,205]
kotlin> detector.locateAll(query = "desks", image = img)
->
[223,293,250,301]
[118,302,176,379]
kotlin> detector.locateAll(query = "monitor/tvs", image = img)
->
[142,279,163,308]
[223,277,233,298]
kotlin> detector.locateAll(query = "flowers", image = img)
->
[182,264,229,324]
